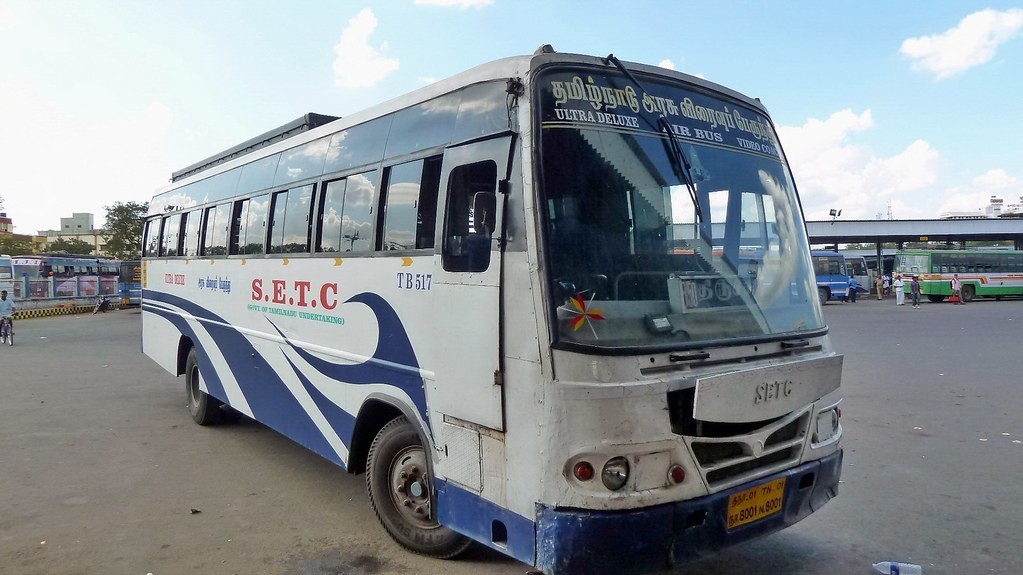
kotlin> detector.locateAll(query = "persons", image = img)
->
[841,272,966,309]
[0,290,16,337]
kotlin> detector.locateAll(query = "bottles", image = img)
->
[872,561,922,575]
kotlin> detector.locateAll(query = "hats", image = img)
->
[954,274,958,276]
[912,276,918,279]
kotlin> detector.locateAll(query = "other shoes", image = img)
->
[912,306,916,309]
[960,302,966,304]
[954,302,958,304]
[901,304,904,305]
[917,306,920,308]
[878,299,882,300]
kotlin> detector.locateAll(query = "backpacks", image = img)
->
[950,278,958,289]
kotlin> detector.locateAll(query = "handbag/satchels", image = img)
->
[949,294,960,302]
[844,288,849,296]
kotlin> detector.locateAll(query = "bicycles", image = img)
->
[0,312,17,346]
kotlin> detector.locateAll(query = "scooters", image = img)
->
[93,293,111,315]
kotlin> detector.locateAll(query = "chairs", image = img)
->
[932,266,967,273]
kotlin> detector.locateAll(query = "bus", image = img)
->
[737,248,848,305]
[844,256,872,296]
[848,249,899,286]
[141,44,844,575]
[12,251,141,307]
[0,255,16,297]
[892,245,1023,303]
